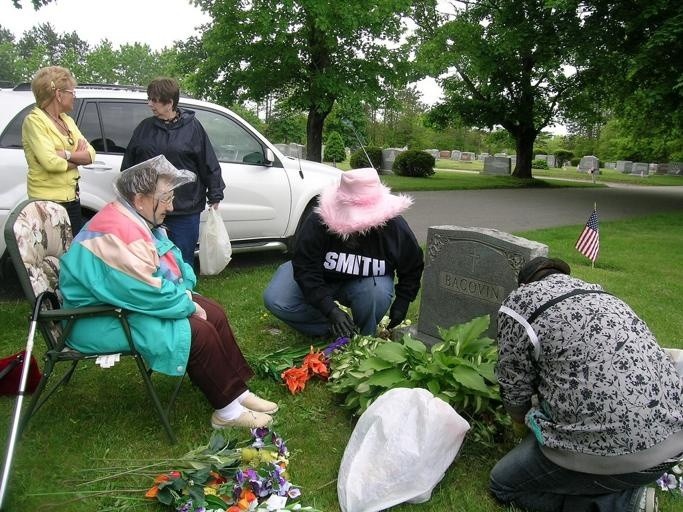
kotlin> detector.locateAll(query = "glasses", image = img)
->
[147,195,176,206]
[148,97,164,102]
[52,88,76,95]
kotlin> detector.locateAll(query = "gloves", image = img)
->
[387,295,409,329]
[185,289,207,321]
[327,307,355,337]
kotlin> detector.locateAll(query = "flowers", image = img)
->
[25,427,320,512]
[654,460,683,497]
[242,330,329,395]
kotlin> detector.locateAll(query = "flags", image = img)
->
[575,209,600,262]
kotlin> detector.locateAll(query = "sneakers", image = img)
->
[211,392,279,429]
[638,487,658,512]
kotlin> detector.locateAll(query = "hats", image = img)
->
[319,168,412,235]
[518,257,570,287]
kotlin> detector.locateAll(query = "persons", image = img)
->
[263,168,424,337]
[23,67,96,239]
[120,79,227,272]
[489,256,683,512]
[59,155,277,429]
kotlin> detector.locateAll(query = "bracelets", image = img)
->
[65,150,71,161]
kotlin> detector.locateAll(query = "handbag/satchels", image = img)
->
[0,351,42,396]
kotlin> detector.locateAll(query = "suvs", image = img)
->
[0,79,349,289]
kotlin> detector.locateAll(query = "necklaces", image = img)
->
[43,108,74,145]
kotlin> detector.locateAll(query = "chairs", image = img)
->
[3,197,186,444]
